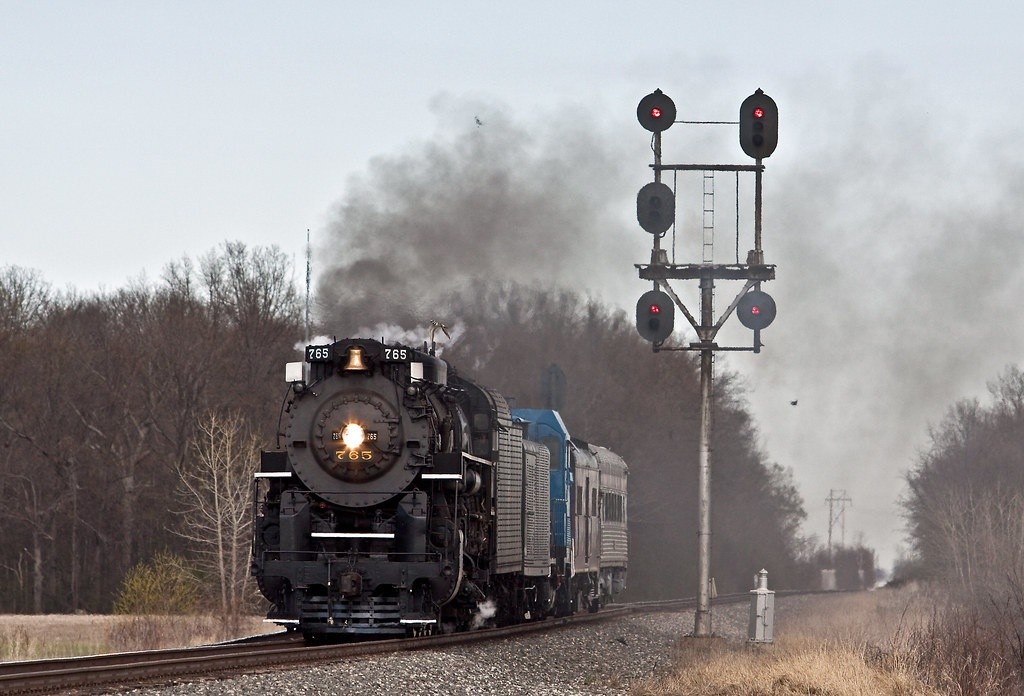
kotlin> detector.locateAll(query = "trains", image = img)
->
[250,324,627,640]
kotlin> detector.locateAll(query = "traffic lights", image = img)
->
[636,86,779,342]
